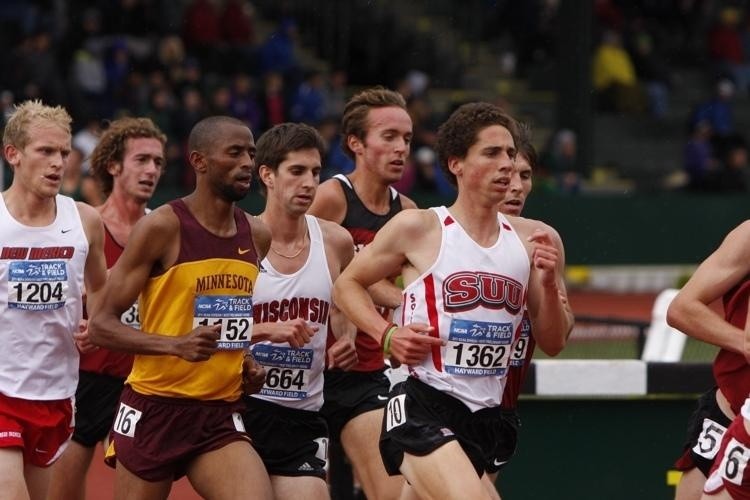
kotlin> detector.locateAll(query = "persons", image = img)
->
[330,101,574,499]
[667,217,750,500]
[499,118,568,429]
[50,117,167,499]
[306,86,420,500]
[243,123,359,499]
[698,393,750,500]
[87,114,274,498]
[1,1,749,208]
[1,98,107,500]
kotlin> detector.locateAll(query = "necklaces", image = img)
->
[255,212,309,259]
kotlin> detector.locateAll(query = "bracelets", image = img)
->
[381,322,399,353]
